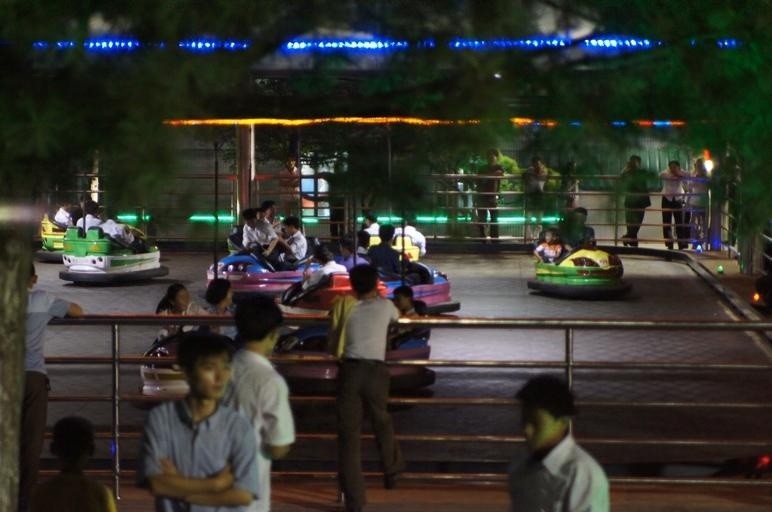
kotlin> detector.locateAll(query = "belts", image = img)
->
[345,358,382,364]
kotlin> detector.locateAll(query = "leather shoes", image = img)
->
[385,472,399,490]
